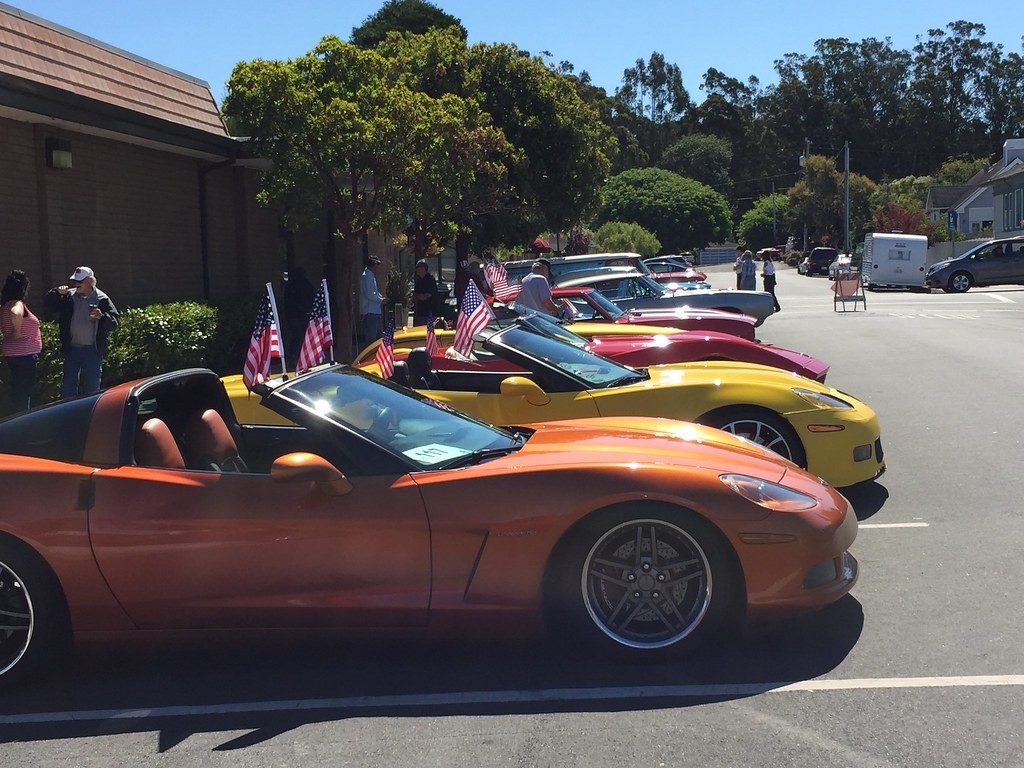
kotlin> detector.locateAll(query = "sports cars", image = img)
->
[1,358,861,694]
[350,251,833,386]
[220,322,887,499]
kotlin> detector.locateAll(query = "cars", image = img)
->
[797,256,810,275]
[828,253,860,281]
[925,235,1024,293]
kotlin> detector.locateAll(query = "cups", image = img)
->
[89,304,97,314]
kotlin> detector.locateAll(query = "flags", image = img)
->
[374,318,395,380]
[454,280,496,359]
[243,289,284,401]
[482,253,522,300]
[424,313,438,356]
[296,282,334,372]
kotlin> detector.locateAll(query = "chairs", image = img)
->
[185,409,249,472]
[407,348,442,392]
[133,417,187,471]
[389,360,417,390]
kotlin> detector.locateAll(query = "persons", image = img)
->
[414,261,437,325]
[522,260,562,318]
[44,266,120,399]
[0,270,43,414]
[733,250,756,290]
[761,251,781,312]
[359,255,385,345]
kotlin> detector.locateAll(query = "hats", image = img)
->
[760,251,770,256]
[743,250,753,257]
[534,259,553,278]
[69,266,94,281]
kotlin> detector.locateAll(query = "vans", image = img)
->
[805,247,839,277]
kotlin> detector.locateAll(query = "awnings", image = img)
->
[532,238,551,254]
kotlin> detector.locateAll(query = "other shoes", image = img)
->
[775,305,780,313]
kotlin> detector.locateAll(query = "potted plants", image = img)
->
[385,271,414,328]
